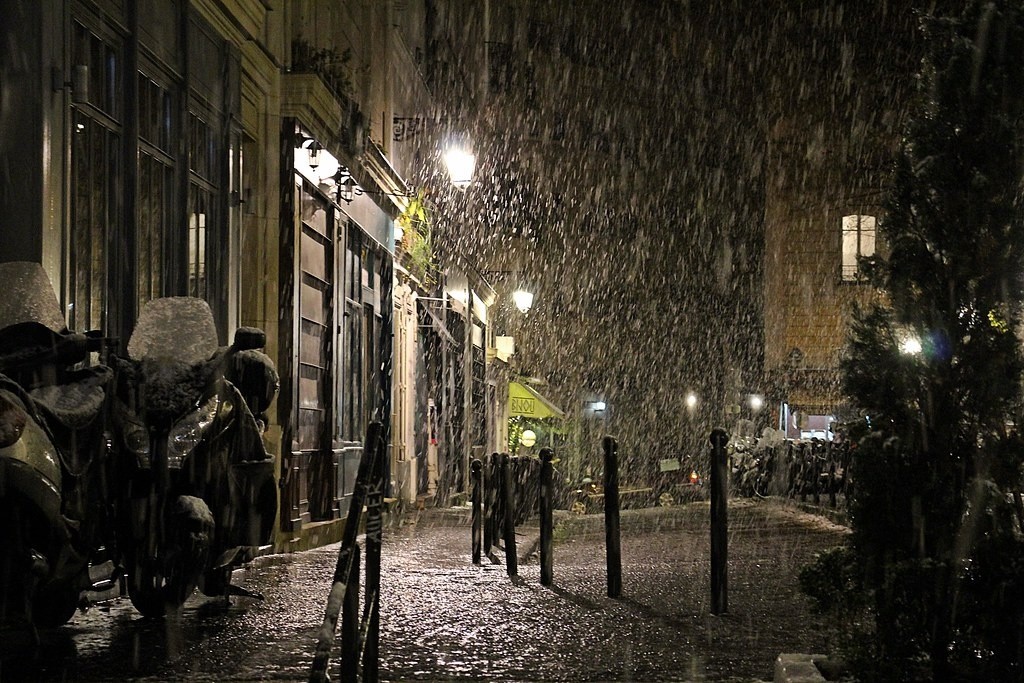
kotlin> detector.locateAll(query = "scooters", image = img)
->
[0,260,123,672]
[86,293,281,617]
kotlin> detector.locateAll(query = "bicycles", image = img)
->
[727,426,858,498]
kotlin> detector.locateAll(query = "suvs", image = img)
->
[564,448,702,517]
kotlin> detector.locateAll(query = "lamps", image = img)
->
[336,165,357,205]
[393,116,476,193]
[295,132,323,172]
[478,268,534,314]
